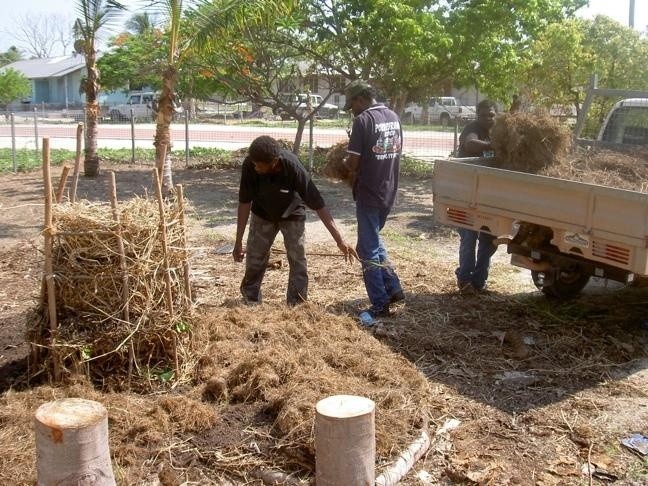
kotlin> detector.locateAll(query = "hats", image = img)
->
[342,82,372,111]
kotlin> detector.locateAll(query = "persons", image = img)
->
[454,94,522,297]
[233,135,359,309]
[341,78,405,325]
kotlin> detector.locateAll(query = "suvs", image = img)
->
[276,92,340,118]
[108,92,184,123]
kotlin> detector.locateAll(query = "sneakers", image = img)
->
[456,274,485,294]
[358,292,405,317]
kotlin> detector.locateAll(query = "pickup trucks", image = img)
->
[432,96,648,308]
[403,96,477,126]
